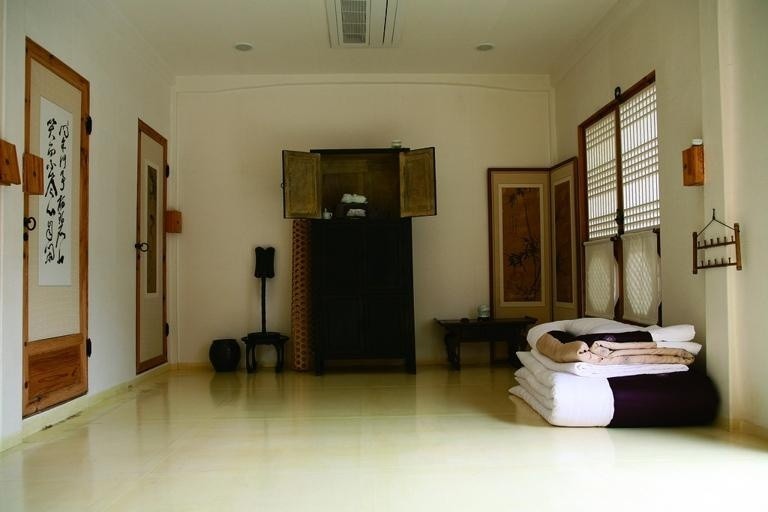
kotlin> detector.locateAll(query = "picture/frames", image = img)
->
[487,156,582,325]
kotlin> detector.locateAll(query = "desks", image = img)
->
[241,335,290,373]
[437,316,538,370]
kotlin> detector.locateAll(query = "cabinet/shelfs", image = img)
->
[281,148,437,375]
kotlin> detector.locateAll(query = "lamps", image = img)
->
[249,247,279,339]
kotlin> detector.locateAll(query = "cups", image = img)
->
[322,208,333,219]
[477,304,490,321]
[392,141,401,147]
[691,138,702,146]
